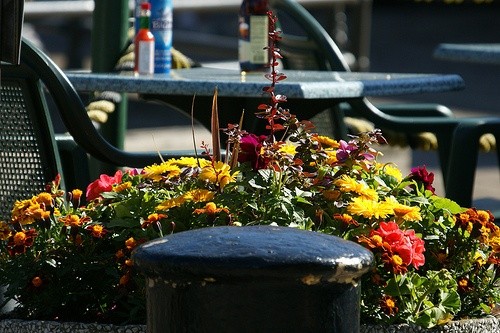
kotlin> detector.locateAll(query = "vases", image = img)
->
[0,318,499,333]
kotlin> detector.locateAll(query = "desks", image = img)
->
[63,64,467,152]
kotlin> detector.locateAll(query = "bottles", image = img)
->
[238,0,271,72]
[133,3,155,77]
[134,0,172,74]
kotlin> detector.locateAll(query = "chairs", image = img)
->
[0,37,226,232]
[269,0,500,207]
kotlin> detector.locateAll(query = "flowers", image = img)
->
[0,135,500,324]
